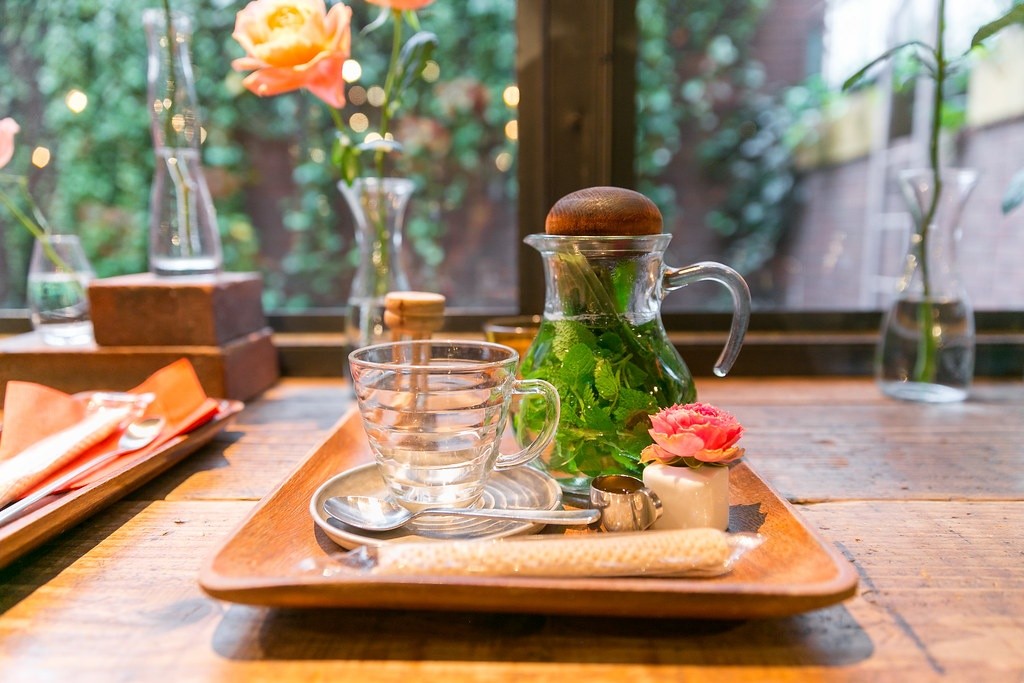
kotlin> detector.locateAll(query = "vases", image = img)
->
[26,234,94,318]
[874,167,981,401]
[642,460,728,532]
[142,8,223,273]
[337,179,414,398]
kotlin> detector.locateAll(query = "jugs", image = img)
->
[514,186,752,497]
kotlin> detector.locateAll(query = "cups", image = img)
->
[347,340,561,513]
[589,474,664,534]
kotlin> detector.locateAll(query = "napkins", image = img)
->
[0,356,219,510]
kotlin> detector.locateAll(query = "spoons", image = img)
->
[322,496,603,531]
[0,417,167,525]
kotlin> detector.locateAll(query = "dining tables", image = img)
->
[0,376,1023,683]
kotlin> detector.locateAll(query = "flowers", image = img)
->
[0,116,75,272]
[231,0,438,178]
[636,403,745,468]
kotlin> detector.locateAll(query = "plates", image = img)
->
[310,462,564,551]
